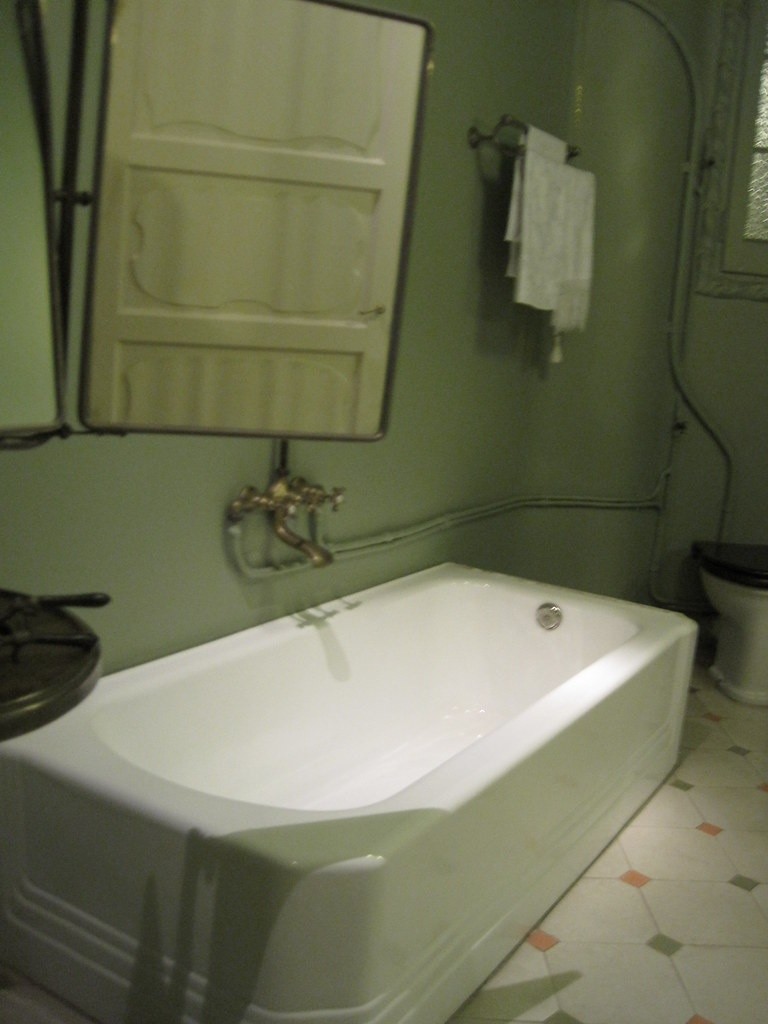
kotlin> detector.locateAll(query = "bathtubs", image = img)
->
[0,562,698,1024]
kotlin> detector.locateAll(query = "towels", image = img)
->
[504,121,596,365]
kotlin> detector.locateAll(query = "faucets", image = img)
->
[227,477,345,568]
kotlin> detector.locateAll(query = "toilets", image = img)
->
[693,540,768,705]
